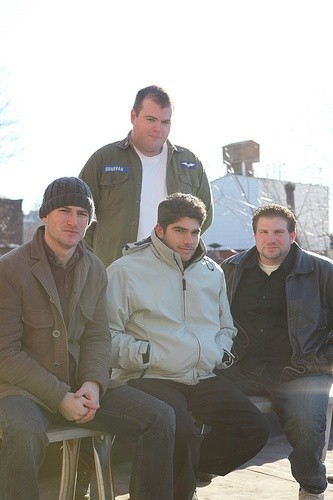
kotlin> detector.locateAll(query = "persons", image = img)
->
[218,204,333,500]
[104,192,271,500]
[0,177,176,500]
[79,85,214,268]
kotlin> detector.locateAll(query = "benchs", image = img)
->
[45,389,333,500]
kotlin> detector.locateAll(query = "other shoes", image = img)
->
[298,486,324,500]
[191,489,198,500]
[197,472,211,486]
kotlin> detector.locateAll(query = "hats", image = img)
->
[38,177,93,225]
